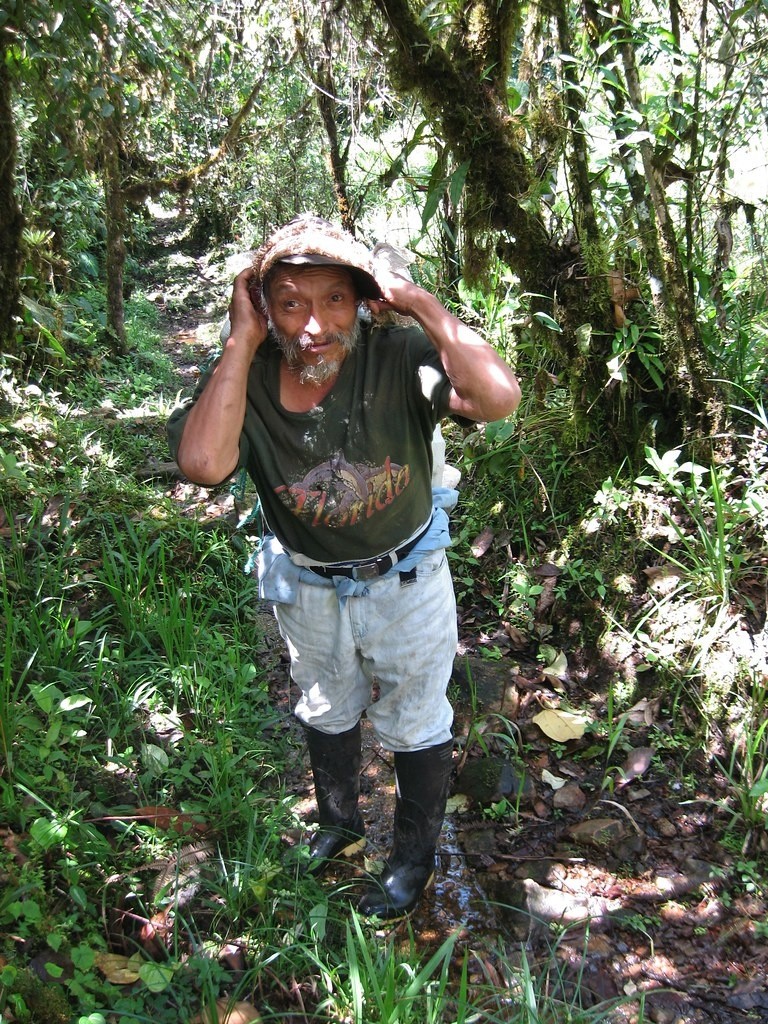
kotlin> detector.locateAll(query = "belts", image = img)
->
[282,514,433,588]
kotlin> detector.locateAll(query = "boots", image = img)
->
[355,727,455,925]
[287,717,366,883]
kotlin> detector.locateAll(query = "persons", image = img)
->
[165,215,522,925]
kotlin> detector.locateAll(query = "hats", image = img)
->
[277,215,381,301]
